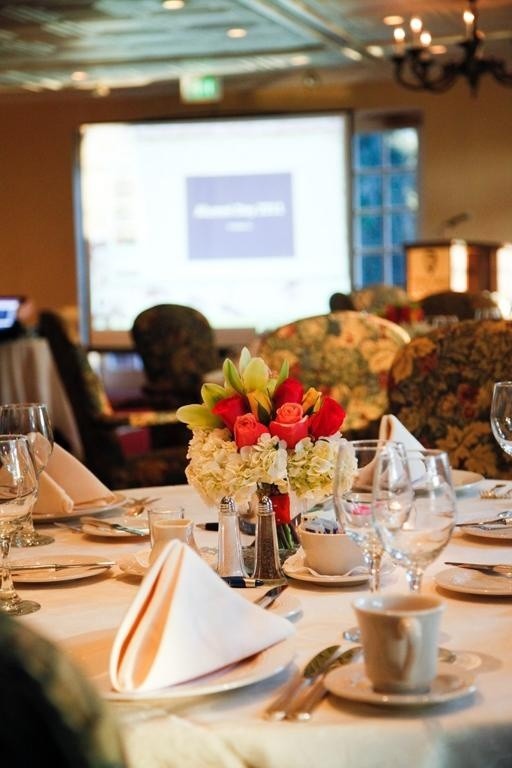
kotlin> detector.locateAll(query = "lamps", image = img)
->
[391,0,511,104]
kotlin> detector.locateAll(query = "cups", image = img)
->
[296,523,359,575]
[349,594,445,691]
[155,519,193,544]
[148,506,184,550]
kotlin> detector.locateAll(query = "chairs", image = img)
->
[254,286,511,493]
[36,305,239,491]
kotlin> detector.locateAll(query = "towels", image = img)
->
[107,538,299,697]
[1,430,117,515]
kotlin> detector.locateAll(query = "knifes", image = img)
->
[256,583,290,613]
[444,558,509,579]
[263,642,362,722]
[80,513,145,535]
[10,560,117,571]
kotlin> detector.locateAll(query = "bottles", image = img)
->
[216,495,249,582]
[252,495,288,583]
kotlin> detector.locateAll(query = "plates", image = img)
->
[430,564,511,600]
[346,468,482,493]
[99,640,294,696]
[84,517,148,538]
[281,552,371,586]
[324,668,479,707]
[462,514,511,542]
[271,593,308,621]
[10,554,112,584]
[32,495,129,522]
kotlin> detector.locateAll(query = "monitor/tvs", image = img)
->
[0,296,26,334]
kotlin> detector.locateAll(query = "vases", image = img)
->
[249,480,303,549]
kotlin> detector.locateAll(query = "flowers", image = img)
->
[176,343,357,521]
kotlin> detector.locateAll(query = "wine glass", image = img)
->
[331,441,411,642]
[0,433,42,618]
[372,446,453,645]
[490,382,511,498]
[2,403,54,549]
